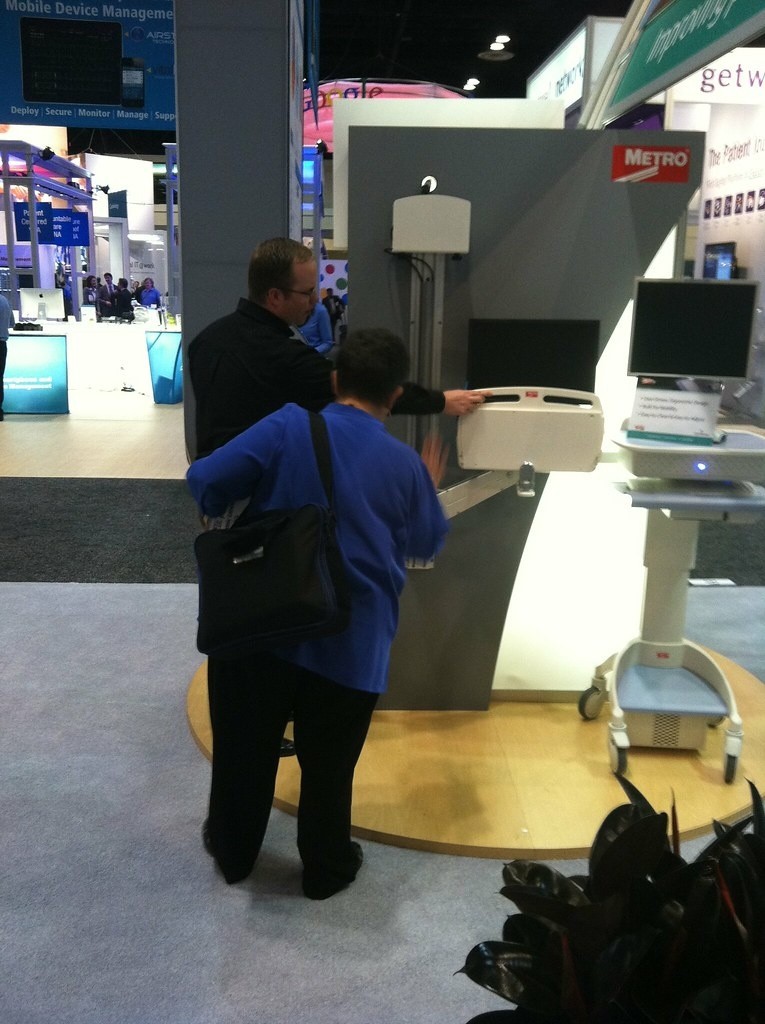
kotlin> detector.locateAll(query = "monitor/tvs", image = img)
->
[462,318,599,406]
[624,274,757,398]
[702,240,739,281]
[19,287,66,322]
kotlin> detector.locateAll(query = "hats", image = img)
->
[60,283,65,286]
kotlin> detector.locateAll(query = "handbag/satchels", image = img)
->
[121,311,135,321]
[194,503,351,660]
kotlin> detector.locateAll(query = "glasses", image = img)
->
[279,286,316,305]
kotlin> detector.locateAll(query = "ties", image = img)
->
[108,286,114,298]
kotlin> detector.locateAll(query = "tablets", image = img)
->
[20,16,123,107]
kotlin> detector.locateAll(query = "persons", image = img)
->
[131,277,161,309]
[55,274,71,301]
[188,327,447,901]
[83,272,131,318]
[322,288,345,345]
[0,295,15,422]
[293,290,333,354]
[189,236,483,756]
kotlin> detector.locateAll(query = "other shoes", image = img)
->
[333,340,337,345]
[202,818,225,877]
[352,841,364,869]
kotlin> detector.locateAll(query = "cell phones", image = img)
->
[119,58,146,109]
